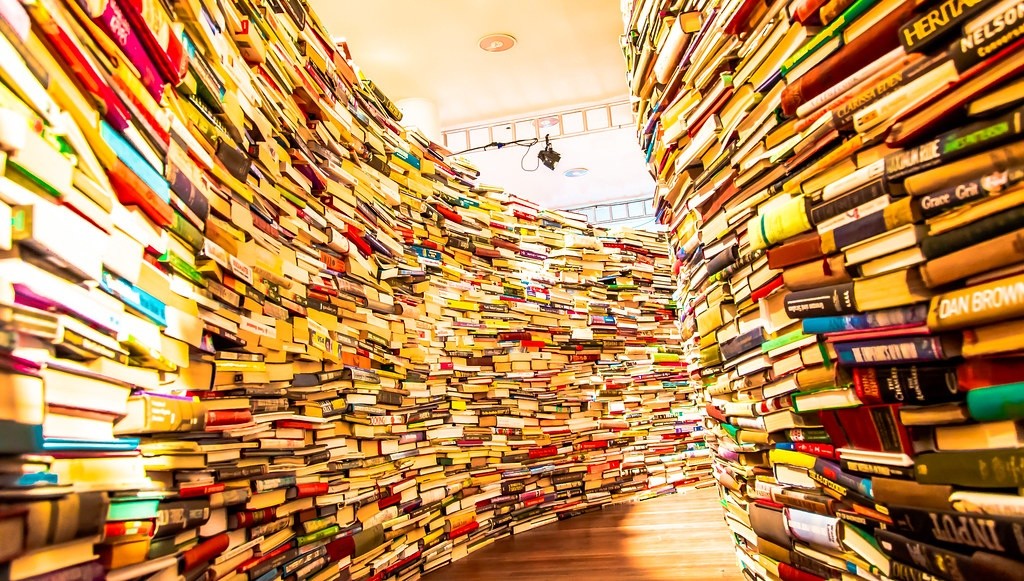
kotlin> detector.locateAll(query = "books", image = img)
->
[0,0,1024,581]
